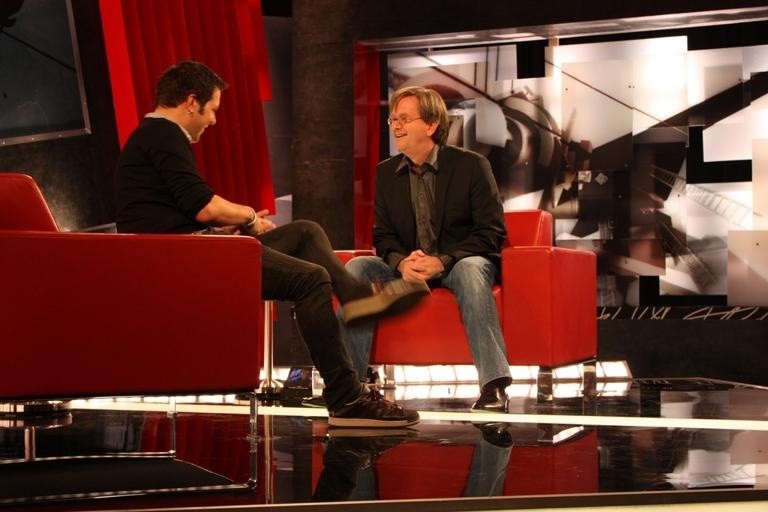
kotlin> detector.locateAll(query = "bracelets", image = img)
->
[241,205,257,228]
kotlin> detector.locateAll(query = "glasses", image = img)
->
[388,115,422,124]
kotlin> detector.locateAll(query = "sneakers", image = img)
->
[341,280,431,327]
[325,428,421,463]
[327,389,420,428]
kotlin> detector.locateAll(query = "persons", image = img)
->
[308,428,421,502]
[300,84,514,415]
[348,419,516,500]
[113,61,433,428]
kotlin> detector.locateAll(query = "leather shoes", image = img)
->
[474,422,512,447]
[302,392,325,408]
[470,386,510,413]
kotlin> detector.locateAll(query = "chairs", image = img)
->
[1,418,265,511]
[311,431,611,495]
[329,209,598,402]
[0,172,264,419]
